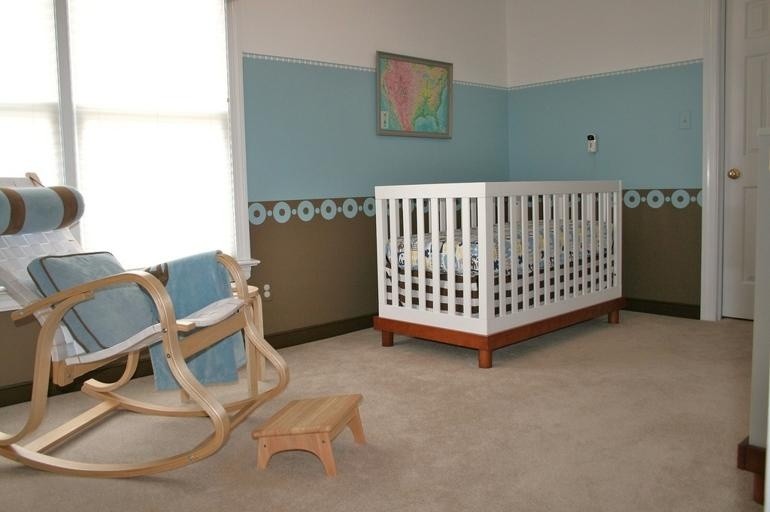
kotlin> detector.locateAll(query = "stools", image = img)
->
[180,286,266,403]
[252,393,363,477]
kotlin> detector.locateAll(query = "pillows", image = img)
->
[26,250,159,354]
[0,185,84,236]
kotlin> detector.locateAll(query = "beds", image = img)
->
[373,179,624,368]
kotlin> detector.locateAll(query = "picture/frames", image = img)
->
[376,50,453,139]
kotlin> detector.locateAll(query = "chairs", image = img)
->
[0,172,289,479]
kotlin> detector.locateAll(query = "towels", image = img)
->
[137,250,247,391]
